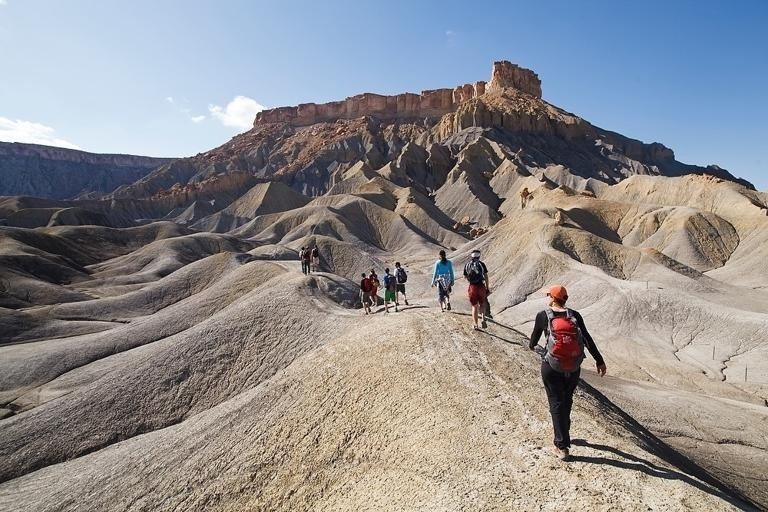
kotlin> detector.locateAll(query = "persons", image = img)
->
[302,246,311,276]
[382,267,398,313]
[368,268,379,308]
[358,272,372,315]
[393,261,409,306]
[298,246,305,274]
[462,249,492,332]
[431,249,453,312]
[527,284,607,461]
[311,245,320,272]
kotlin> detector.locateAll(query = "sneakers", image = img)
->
[553,448,568,461]
[447,303,451,310]
[481,321,487,328]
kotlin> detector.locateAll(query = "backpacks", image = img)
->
[538,308,586,372]
[365,275,380,290]
[388,275,396,289]
[398,269,407,283]
[468,261,482,283]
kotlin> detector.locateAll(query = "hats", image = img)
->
[547,286,567,301]
